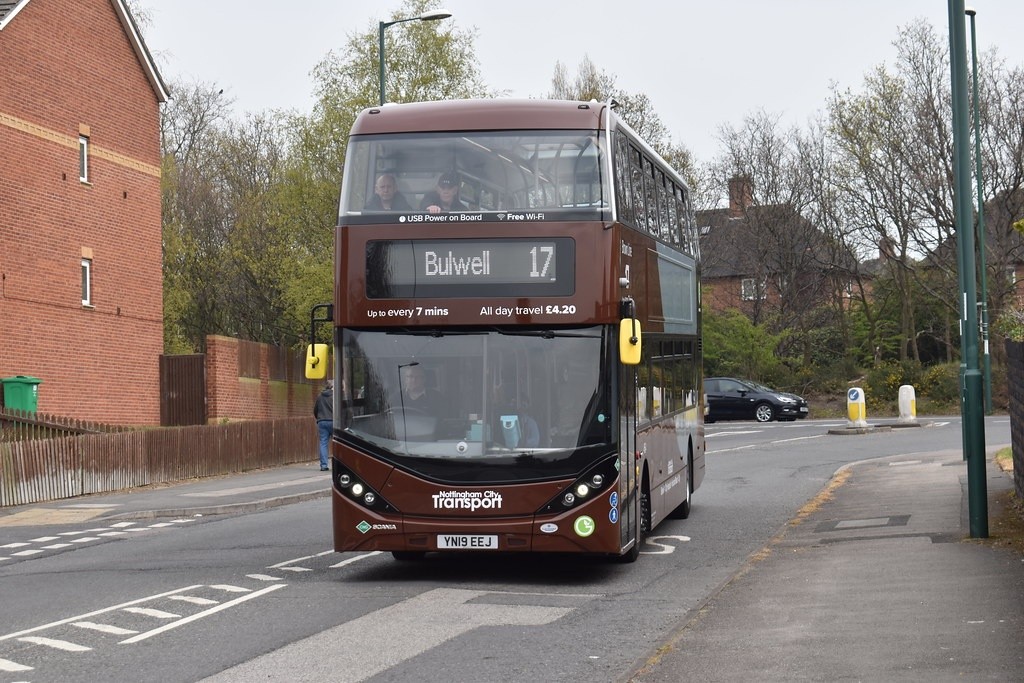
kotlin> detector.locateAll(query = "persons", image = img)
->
[378,364,454,442]
[313,380,334,471]
[363,175,414,211]
[419,173,470,214]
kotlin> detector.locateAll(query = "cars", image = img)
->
[702,377,809,424]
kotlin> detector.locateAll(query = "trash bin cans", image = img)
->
[0,376,43,427]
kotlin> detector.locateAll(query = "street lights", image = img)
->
[379,8,453,105]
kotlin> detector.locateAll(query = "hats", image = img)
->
[439,173,461,188]
[325,380,333,389]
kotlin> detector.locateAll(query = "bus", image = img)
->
[306,97,707,566]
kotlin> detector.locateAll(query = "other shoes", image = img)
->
[321,468,329,471]
